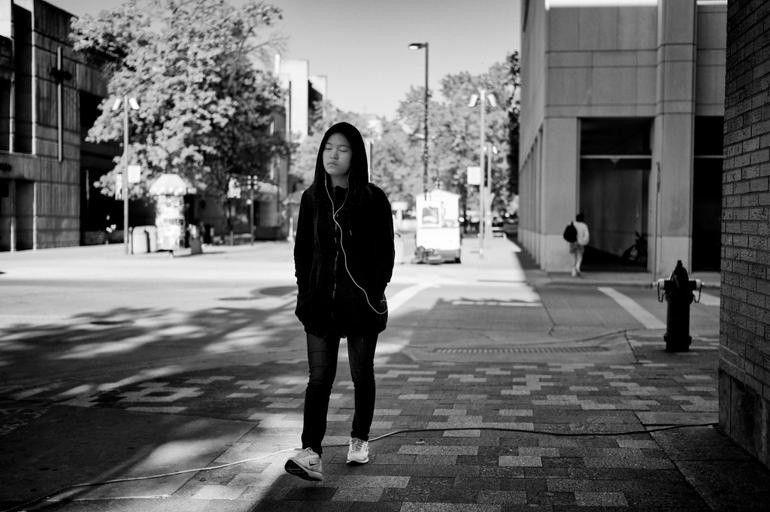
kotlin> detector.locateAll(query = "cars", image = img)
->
[387,208,519,240]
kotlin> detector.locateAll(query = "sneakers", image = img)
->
[285,447,323,481]
[346,437,370,466]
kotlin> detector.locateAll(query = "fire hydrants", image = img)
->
[648,258,705,353]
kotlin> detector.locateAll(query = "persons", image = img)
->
[568,212,589,279]
[282,121,397,481]
[101,214,115,244]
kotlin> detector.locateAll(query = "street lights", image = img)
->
[466,87,499,245]
[111,94,139,254]
[407,39,430,199]
